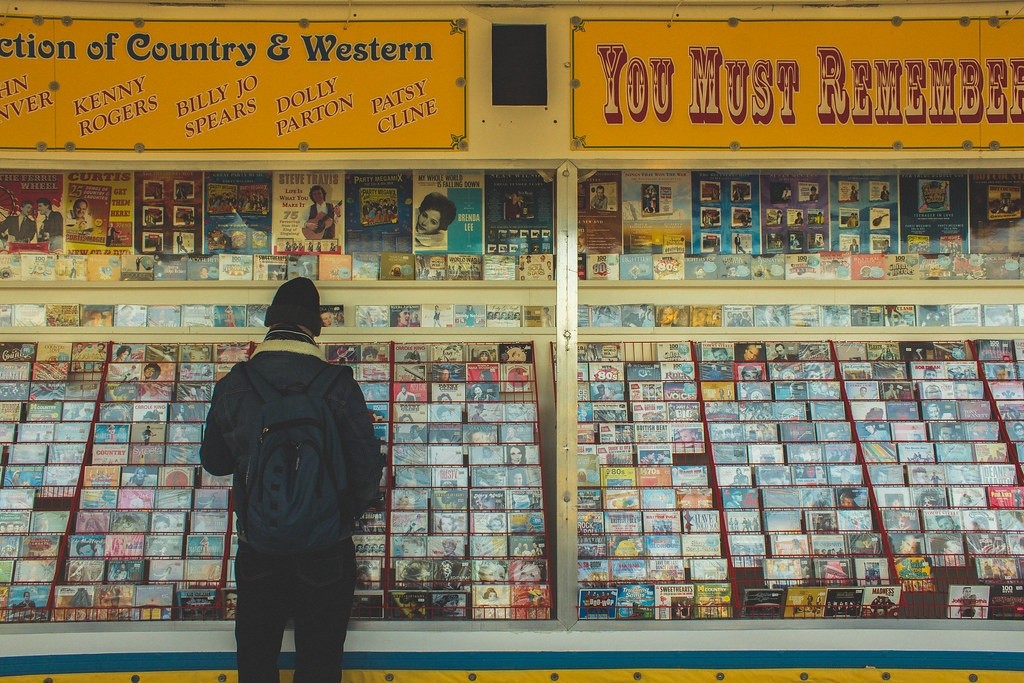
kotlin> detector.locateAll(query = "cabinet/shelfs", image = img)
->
[0,153,1024,683]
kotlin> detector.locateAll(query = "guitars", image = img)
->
[302,200,342,239]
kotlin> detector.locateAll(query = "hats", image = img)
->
[264,275,323,337]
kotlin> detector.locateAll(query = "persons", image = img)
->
[0,185,1024,619]
[200,277,383,683]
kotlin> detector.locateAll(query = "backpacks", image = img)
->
[224,356,354,560]
